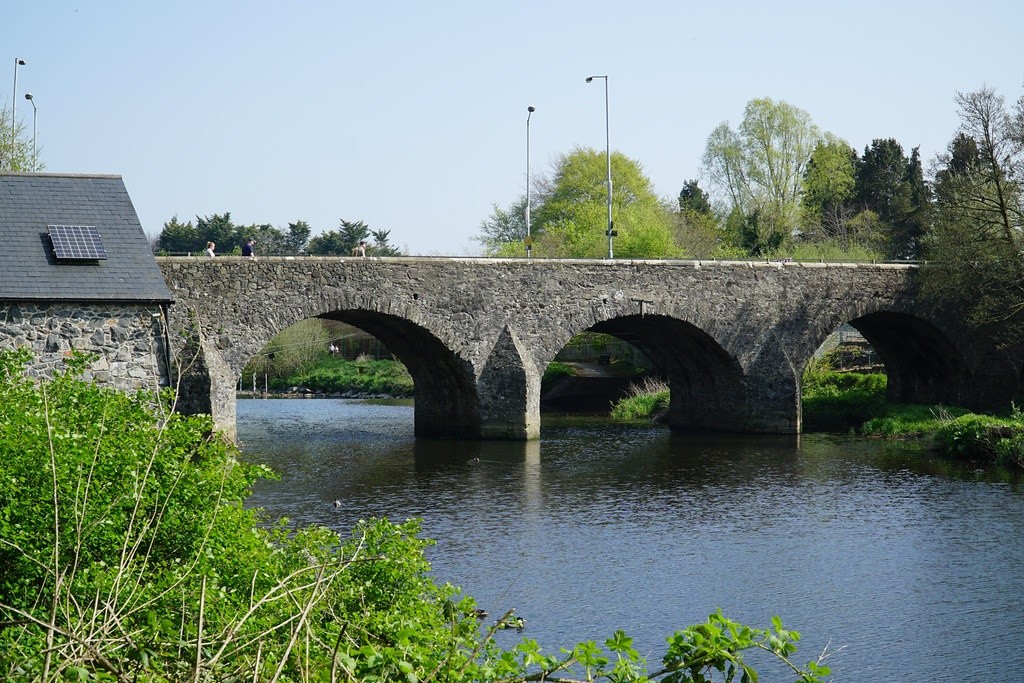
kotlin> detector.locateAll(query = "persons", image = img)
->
[351,241,368,257]
[329,342,339,355]
[242,238,256,256]
[206,241,215,256]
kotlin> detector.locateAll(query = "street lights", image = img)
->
[11,58,25,158]
[24,92,37,171]
[526,104,535,257]
[586,75,613,259]
